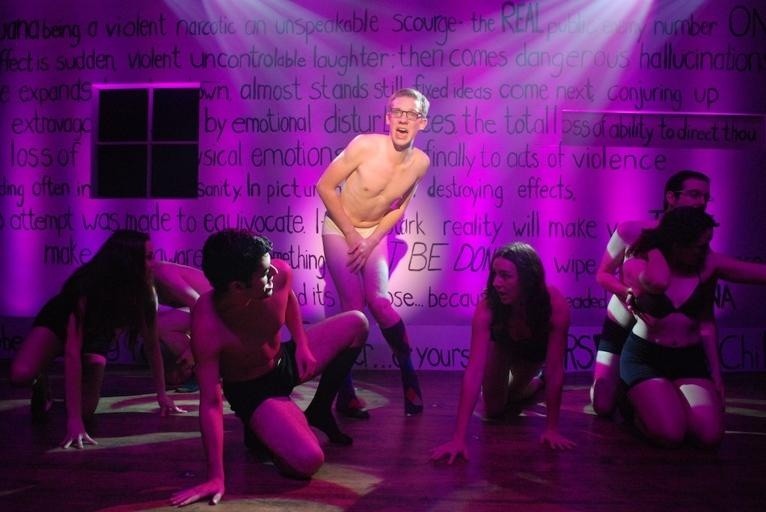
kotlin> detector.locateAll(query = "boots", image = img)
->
[336,373,368,420]
[382,320,425,417]
[303,347,361,446]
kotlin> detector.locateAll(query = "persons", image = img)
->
[9,229,188,451]
[428,241,579,466]
[166,227,369,509]
[617,206,766,448]
[313,88,429,421]
[143,246,215,386]
[590,169,715,418]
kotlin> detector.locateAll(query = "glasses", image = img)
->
[387,109,424,122]
